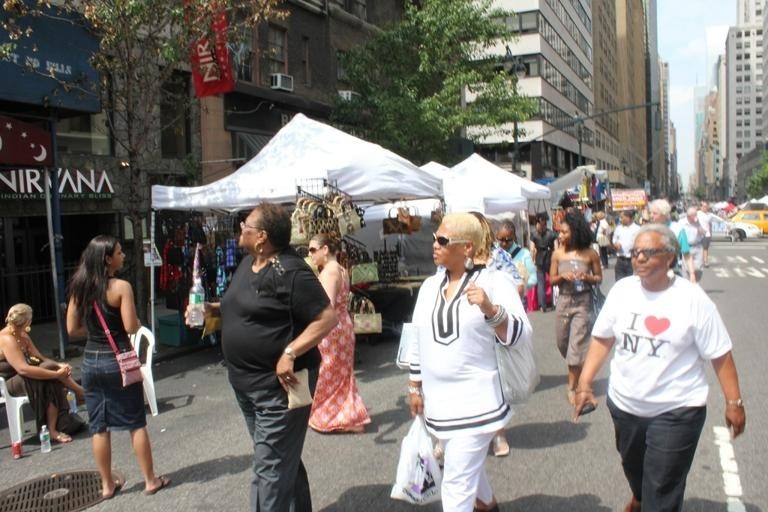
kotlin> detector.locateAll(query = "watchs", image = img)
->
[726,400,743,407]
[283,348,297,359]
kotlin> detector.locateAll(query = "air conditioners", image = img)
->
[339,90,360,101]
[270,72,293,92]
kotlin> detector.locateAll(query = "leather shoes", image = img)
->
[493,436,509,457]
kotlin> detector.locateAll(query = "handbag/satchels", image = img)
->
[383,204,422,234]
[590,283,605,317]
[116,350,143,387]
[350,298,383,335]
[496,334,540,405]
[339,246,400,288]
[289,192,367,245]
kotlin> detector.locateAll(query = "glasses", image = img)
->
[497,237,513,243]
[432,232,470,247]
[629,246,665,256]
[240,222,261,230]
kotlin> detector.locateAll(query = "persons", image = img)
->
[435,210,525,466]
[405,213,532,512]
[532,200,736,404]
[308,231,372,433]
[574,224,746,512]
[185,203,339,511]
[0,303,85,443]
[66,235,168,496]
[493,222,538,289]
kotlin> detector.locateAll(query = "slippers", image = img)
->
[102,479,121,498]
[55,432,72,442]
[143,476,169,496]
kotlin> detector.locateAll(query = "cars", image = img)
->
[728,210,768,236]
[674,209,762,243]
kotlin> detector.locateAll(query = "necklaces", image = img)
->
[11,329,30,357]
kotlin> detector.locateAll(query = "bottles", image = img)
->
[39,425,53,454]
[66,390,80,414]
[185,276,207,327]
[569,260,585,294]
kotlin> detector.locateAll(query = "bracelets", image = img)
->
[486,305,506,328]
[576,389,594,393]
[408,387,423,393]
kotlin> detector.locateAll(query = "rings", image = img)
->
[286,376,292,381]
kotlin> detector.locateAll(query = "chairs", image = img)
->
[0,376,30,445]
[128,326,159,417]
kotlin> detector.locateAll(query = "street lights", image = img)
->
[569,111,585,168]
[502,44,527,177]
